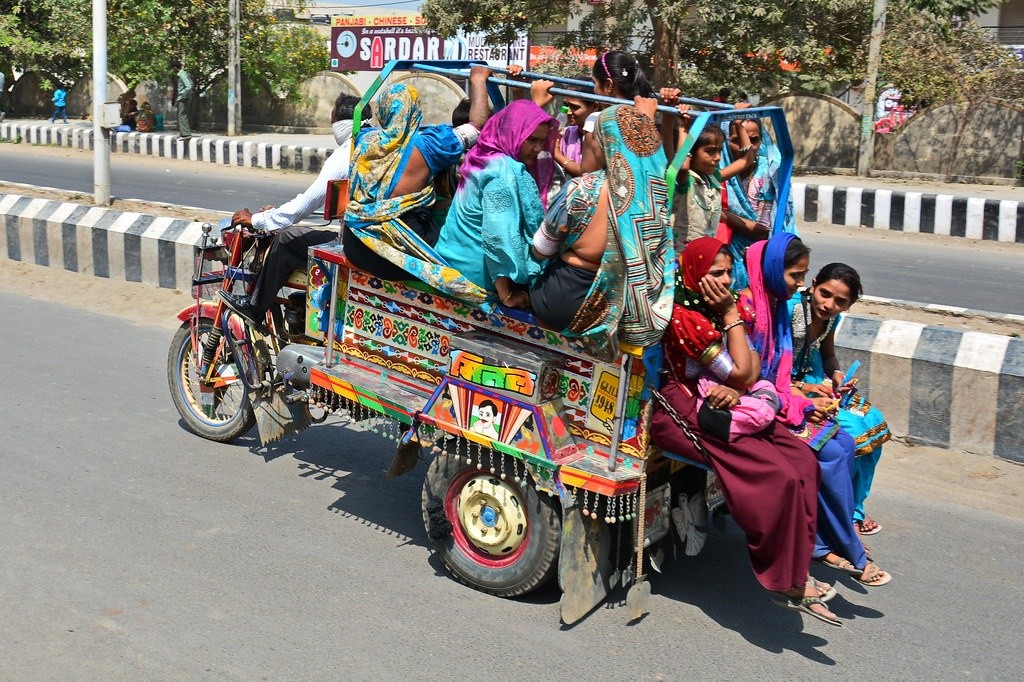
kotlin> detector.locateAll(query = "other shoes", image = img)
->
[177,135,192,140]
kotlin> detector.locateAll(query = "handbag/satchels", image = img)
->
[694,372,779,442]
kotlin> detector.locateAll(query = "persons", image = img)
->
[650,233,891,624]
[113,89,162,133]
[343,51,800,345]
[52,85,67,124]
[213,94,378,325]
[172,61,193,141]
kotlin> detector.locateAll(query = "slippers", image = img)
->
[859,515,883,535]
[848,559,892,587]
[812,552,864,574]
[770,576,839,609]
[858,542,874,561]
[776,589,843,628]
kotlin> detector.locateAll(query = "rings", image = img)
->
[726,395,733,400]
[854,379,859,384]
[704,296,710,301]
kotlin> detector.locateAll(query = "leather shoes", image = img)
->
[215,288,266,329]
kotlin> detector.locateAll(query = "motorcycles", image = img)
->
[167,59,796,598]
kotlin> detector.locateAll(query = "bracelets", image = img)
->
[723,319,744,331]
[790,383,796,387]
[563,159,573,168]
[739,144,753,152]
[830,369,845,377]
[502,292,512,302]
[797,382,805,390]
